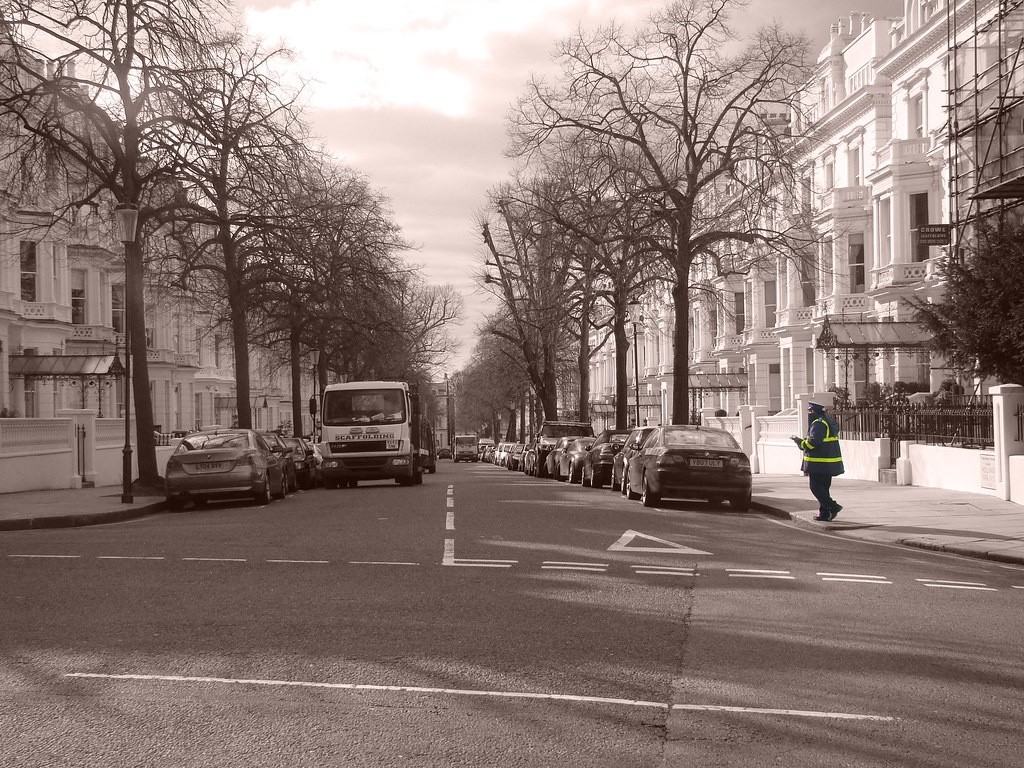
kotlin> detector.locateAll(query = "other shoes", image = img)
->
[813,515,830,521]
[829,504,842,521]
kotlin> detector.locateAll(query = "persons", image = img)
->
[789,401,845,521]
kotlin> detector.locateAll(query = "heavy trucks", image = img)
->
[308,380,437,489]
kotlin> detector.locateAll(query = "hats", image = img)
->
[807,402,824,410]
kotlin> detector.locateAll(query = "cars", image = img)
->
[480,420,594,478]
[282,437,318,490]
[164,428,286,511]
[626,424,753,511]
[611,425,660,496]
[543,435,584,480]
[254,428,297,498]
[304,440,323,468]
[438,448,452,460]
[556,436,596,484]
[581,429,632,489]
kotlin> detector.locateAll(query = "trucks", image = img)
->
[451,433,480,463]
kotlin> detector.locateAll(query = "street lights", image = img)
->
[309,344,320,443]
[112,196,141,505]
[628,293,643,427]
[497,411,503,441]
